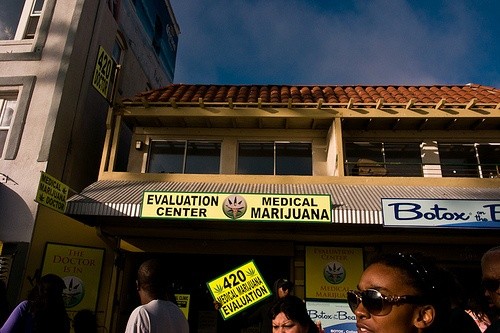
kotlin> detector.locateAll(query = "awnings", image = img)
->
[63,180,500,227]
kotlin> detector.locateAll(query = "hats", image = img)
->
[278,279,293,287]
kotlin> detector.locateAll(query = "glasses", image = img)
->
[480,279,500,292]
[347,289,423,316]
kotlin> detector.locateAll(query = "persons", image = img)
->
[271,295,319,333]
[463,246,500,333]
[0,273,70,333]
[124,260,189,333]
[210,275,293,333]
[347,253,435,333]
